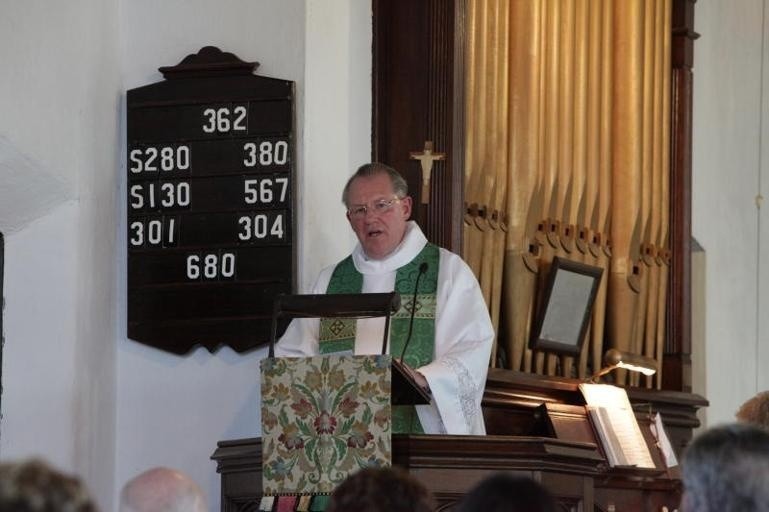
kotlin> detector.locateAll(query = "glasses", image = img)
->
[345,195,405,220]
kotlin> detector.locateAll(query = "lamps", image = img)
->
[586,350,658,386]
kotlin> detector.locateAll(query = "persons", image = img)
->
[0,456,209,512]
[680,391,769,512]
[326,467,567,512]
[274,162,495,435]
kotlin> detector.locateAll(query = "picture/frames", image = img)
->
[530,257,605,357]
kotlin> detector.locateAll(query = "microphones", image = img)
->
[401,262,429,362]
